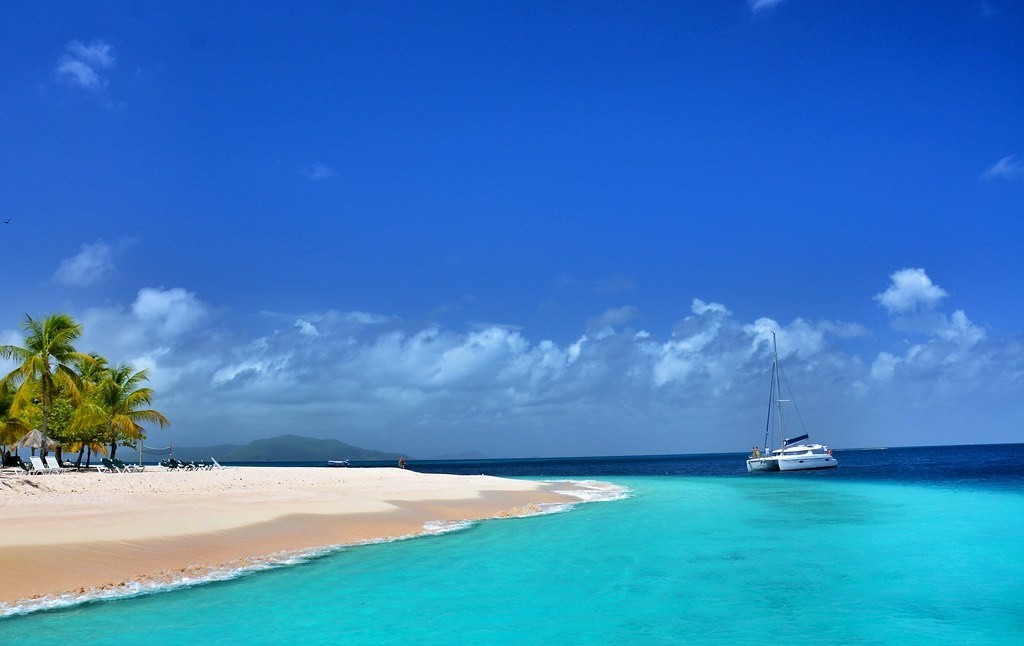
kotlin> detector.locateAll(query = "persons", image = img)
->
[752,447,760,459]
[766,447,769,457]
[398,457,405,469]
[823,445,827,454]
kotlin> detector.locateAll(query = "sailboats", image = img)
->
[746,331,839,471]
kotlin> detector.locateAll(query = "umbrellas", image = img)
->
[11,429,58,460]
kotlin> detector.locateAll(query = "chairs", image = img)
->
[158,457,227,471]
[0,468,25,475]
[96,457,145,474]
[29,455,78,476]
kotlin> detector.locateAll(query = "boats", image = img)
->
[327,460,351,468]
